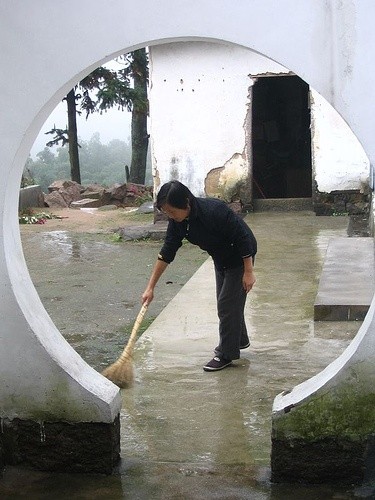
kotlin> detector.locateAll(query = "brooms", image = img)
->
[100,298,149,388]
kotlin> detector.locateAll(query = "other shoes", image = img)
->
[202,355,232,372]
[215,340,249,353]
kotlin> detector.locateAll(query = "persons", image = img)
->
[139,179,260,371]
